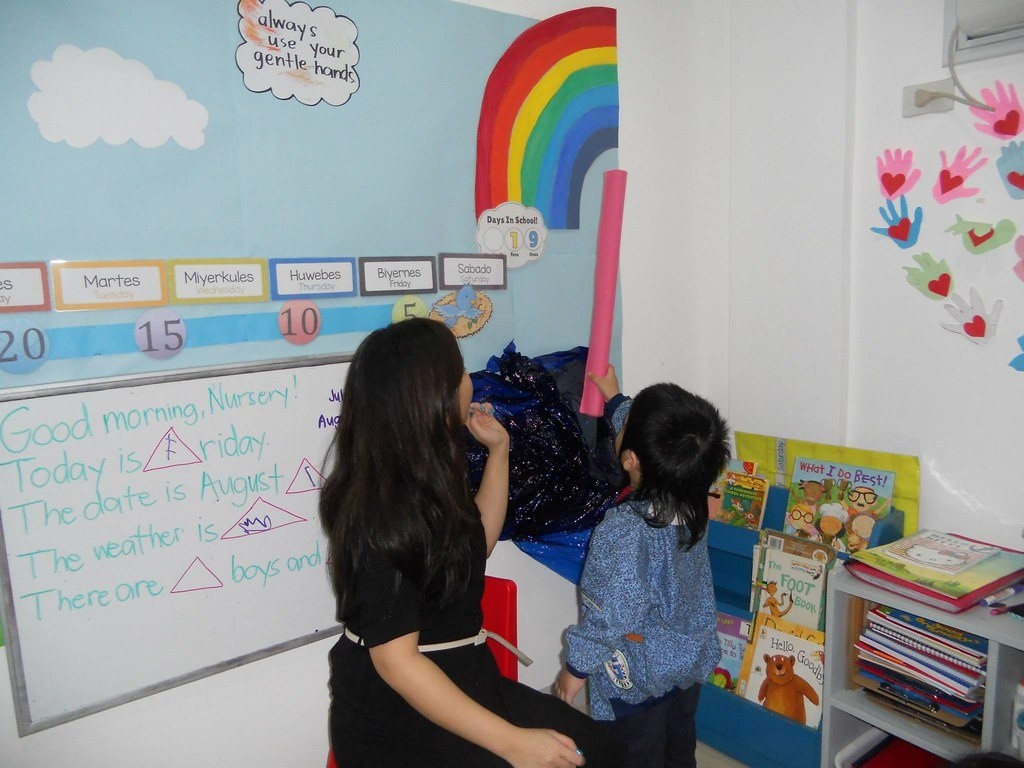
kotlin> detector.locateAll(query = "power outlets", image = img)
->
[900,78,955,118]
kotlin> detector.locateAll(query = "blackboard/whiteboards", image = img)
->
[0,351,356,737]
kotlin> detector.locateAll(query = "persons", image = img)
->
[550,363,732,768]
[317,319,633,768]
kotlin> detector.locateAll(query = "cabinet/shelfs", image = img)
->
[818,566,1024,768]
[695,485,905,768]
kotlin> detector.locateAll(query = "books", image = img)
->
[780,456,896,553]
[850,604,989,742]
[841,528,1024,614]
[706,609,827,728]
[707,458,771,532]
[748,527,838,632]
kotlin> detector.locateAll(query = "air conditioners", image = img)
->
[954,0,1024,39]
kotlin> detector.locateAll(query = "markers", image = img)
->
[979,584,1024,608]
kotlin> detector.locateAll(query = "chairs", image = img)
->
[327,576,518,768]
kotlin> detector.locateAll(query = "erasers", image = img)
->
[991,606,1009,615]
